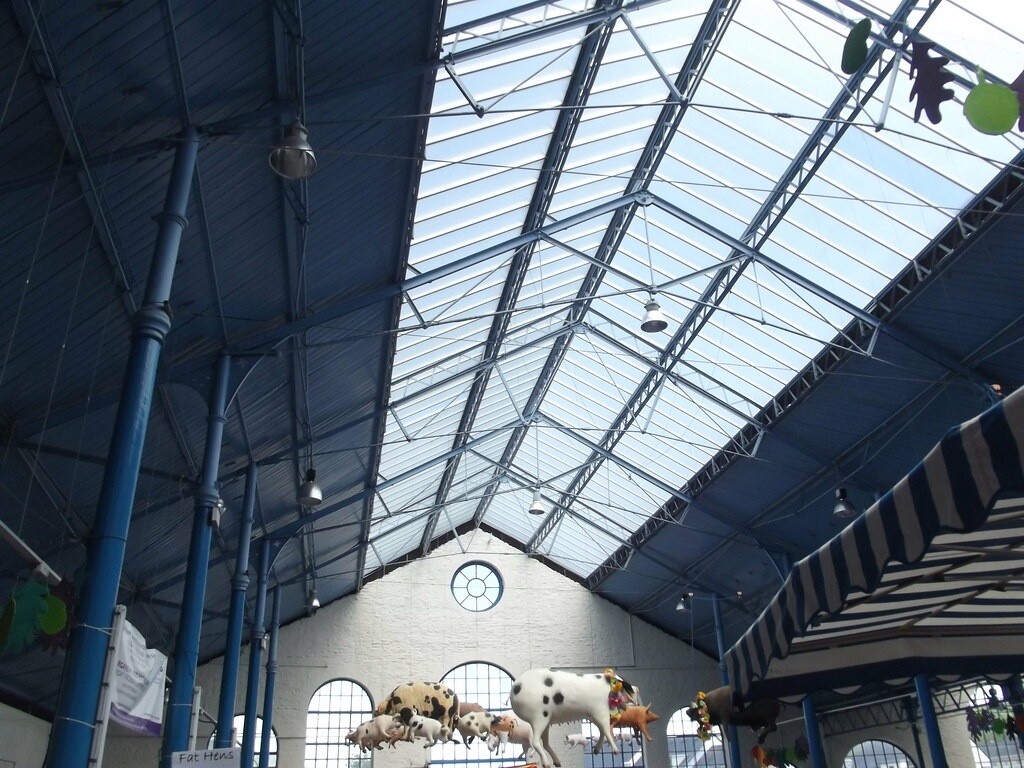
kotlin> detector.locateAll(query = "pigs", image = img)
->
[344,668,787,768]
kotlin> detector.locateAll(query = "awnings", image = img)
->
[722,384,1024,712]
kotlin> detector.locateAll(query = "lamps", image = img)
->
[297,350,324,506]
[528,423,545,515]
[832,488,859,518]
[303,547,321,608]
[640,205,669,333]
[266,100,318,181]
[675,595,692,615]
